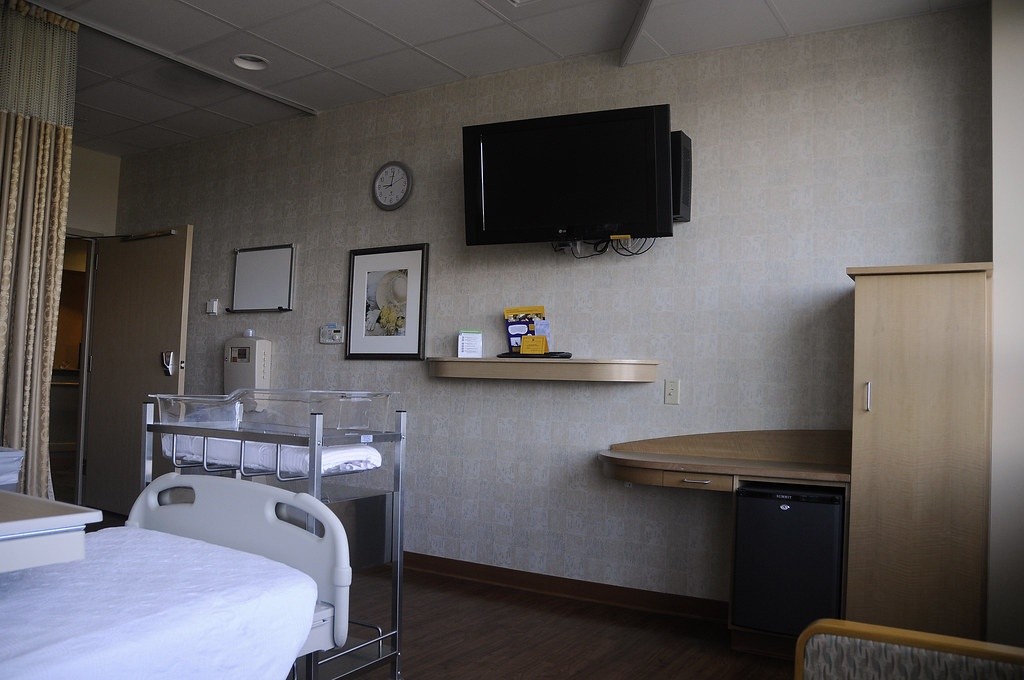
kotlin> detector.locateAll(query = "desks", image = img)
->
[0,491,103,574]
[598,429,851,660]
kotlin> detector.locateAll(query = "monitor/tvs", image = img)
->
[463,103,674,246]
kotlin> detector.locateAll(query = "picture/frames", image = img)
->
[345,243,429,361]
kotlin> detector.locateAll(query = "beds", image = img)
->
[0,472,352,680]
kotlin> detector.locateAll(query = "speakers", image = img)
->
[728,488,846,661]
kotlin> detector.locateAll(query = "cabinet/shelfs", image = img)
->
[846,262,991,642]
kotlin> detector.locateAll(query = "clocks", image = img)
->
[373,161,412,211]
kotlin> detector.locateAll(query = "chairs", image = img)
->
[792,620,1024,680]
